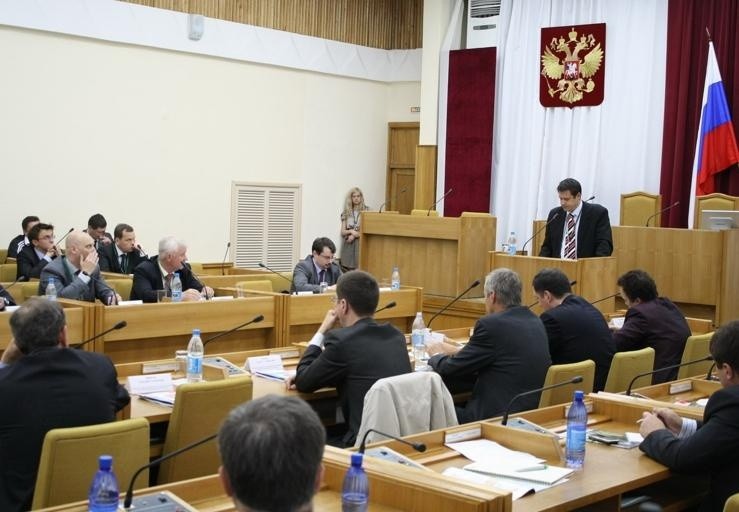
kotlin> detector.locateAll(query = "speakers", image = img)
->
[188,14,204,40]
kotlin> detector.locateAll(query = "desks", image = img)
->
[486,250,617,315]
[533,219,738,331]
[2,249,424,366]
[360,211,497,298]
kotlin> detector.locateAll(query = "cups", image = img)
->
[172,357,186,379]
[157,290,171,303]
[320,282,328,293]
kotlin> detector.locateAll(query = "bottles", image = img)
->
[341,453,369,512]
[88,455,120,512]
[391,266,400,290]
[410,311,427,362]
[172,272,183,301]
[187,329,205,384]
[45,277,60,302]
[564,389,589,468]
[508,232,518,256]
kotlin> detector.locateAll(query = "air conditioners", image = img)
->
[467,0,501,49]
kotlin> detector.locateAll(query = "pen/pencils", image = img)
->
[517,464,548,472]
[636,413,658,424]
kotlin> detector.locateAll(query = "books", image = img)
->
[443,459,575,499]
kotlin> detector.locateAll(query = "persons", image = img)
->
[612,269,691,384]
[639,320,739,511]
[287,272,412,448]
[0,284,16,311]
[8,216,40,257]
[290,237,345,295]
[531,269,619,393]
[38,232,121,305]
[219,395,326,512]
[83,215,113,250]
[15,223,63,281]
[129,236,214,303]
[539,179,613,258]
[427,268,551,424]
[341,188,369,270]
[98,224,148,274]
[0,297,131,512]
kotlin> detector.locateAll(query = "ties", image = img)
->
[166,274,174,297]
[319,270,326,282]
[564,213,576,259]
[120,254,126,274]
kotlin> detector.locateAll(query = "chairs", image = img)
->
[619,192,738,230]
[32,330,739,512]
[412,209,493,218]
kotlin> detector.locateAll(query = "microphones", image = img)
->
[501,376,584,426]
[528,280,576,308]
[83,271,118,305]
[375,301,396,313]
[137,244,150,261]
[203,315,264,345]
[379,186,408,213]
[427,280,480,328]
[359,429,426,454]
[586,196,595,201]
[56,228,74,245]
[522,213,559,255]
[646,201,680,227]
[626,355,713,396]
[259,263,298,295]
[427,189,453,216]
[75,320,127,349]
[180,260,210,300]
[222,242,230,275]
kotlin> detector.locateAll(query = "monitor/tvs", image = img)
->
[702,210,739,231]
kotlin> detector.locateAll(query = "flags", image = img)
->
[688,42,739,231]
